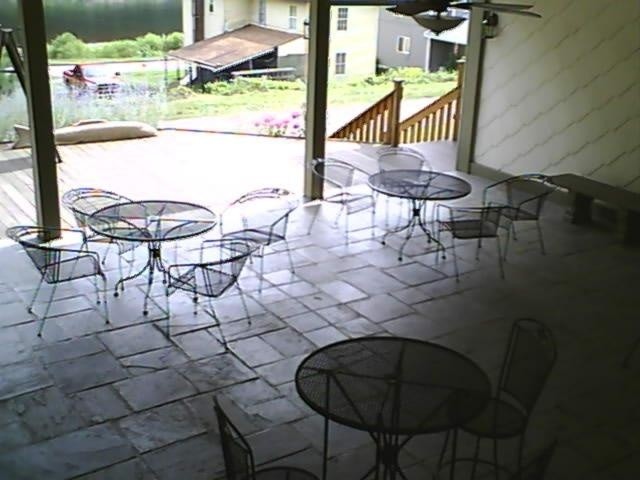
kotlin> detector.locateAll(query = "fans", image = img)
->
[385,0,542,31]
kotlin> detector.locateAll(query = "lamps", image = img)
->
[478,9,499,38]
[411,0,466,37]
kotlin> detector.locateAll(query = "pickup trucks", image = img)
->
[59,62,130,100]
